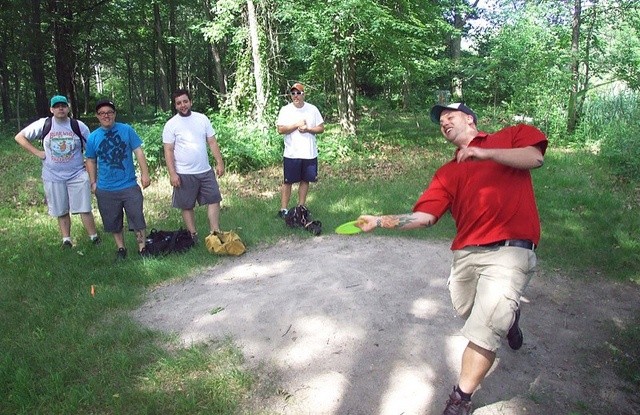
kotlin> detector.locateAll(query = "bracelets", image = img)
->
[377,216,381,228]
[89,181,96,186]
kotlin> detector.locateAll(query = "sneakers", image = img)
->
[192,232,201,244]
[509,311,523,352]
[278,208,288,219]
[60,241,73,252]
[443,385,475,415]
[114,247,128,263]
[91,237,103,247]
[137,247,153,259]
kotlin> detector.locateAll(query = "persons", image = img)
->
[86,102,151,263]
[14,95,100,249]
[354,101,549,415]
[162,90,224,239]
[276,84,324,217]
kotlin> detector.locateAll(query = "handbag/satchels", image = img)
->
[144,227,194,256]
[204,227,246,257]
[284,206,323,236]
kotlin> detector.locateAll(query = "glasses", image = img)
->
[292,90,301,96]
[98,110,115,116]
[54,104,68,108]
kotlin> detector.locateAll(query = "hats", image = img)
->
[431,103,476,125]
[291,83,304,91]
[50,95,68,107]
[97,99,115,112]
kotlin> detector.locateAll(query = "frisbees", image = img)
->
[335,221,366,234]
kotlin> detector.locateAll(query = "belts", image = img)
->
[482,239,537,252]
